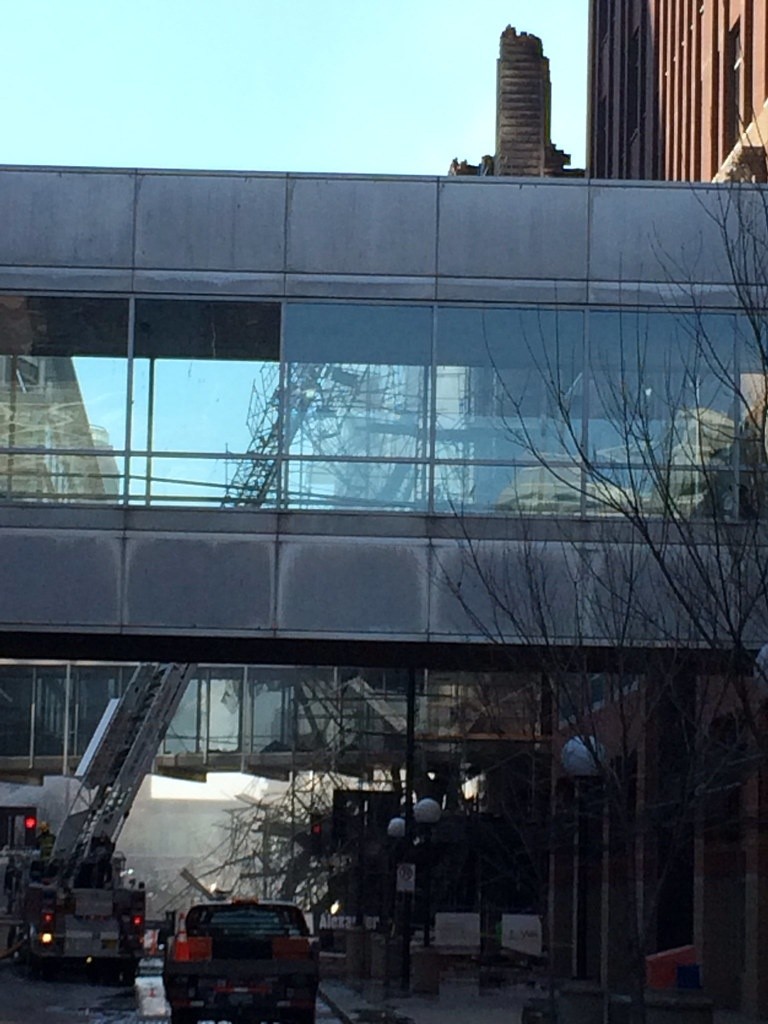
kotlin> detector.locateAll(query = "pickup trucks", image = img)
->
[165,899,320,1024]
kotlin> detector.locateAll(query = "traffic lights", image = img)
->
[311,813,325,859]
[23,808,38,846]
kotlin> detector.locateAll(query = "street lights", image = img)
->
[410,798,439,997]
[560,734,607,1024]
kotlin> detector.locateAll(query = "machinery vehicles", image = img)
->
[0,656,200,983]
